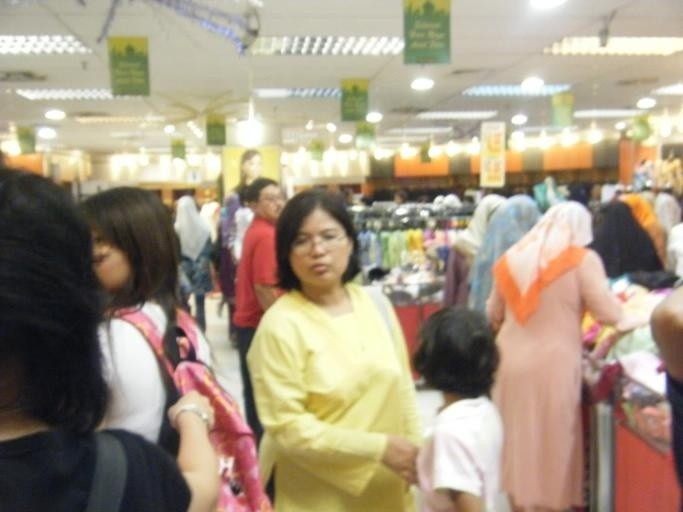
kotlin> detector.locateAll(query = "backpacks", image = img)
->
[108,305,272,511]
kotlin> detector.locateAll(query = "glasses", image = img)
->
[292,227,349,256]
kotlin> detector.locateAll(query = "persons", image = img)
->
[410,306,505,512]
[649,277,683,512]
[173,194,212,334]
[485,198,622,512]
[361,194,375,209]
[201,202,220,242]
[77,187,215,459]
[393,190,409,203]
[0,150,221,512]
[228,186,255,347]
[468,193,544,314]
[245,190,420,512]
[229,176,285,503]
[632,149,683,194]
[444,194,505,308]
[621,193,667,270]
[217,195,239,317]
[232,149,262,195]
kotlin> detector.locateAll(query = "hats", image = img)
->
[369,266,392,282]
